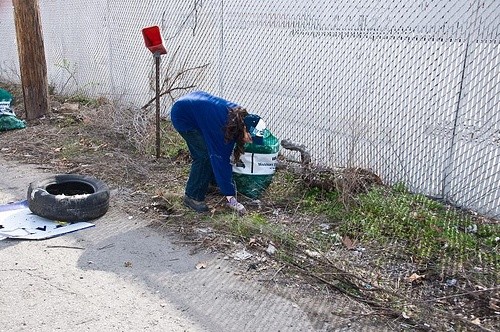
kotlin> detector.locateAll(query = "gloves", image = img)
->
[226,196,246,215]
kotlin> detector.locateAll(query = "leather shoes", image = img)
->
[183,195,209,212]
[209,181,220,190]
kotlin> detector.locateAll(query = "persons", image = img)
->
[171,90,267,212]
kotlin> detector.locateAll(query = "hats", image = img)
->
[242,114,267,145]
